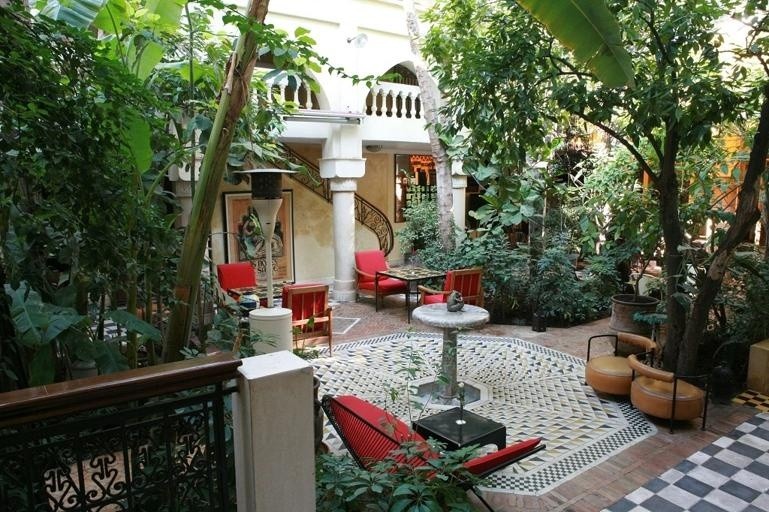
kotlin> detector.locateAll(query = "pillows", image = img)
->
[331,396,441,479]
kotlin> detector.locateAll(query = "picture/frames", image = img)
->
[222,189,295,301]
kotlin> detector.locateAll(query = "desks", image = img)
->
[411,303,490,400]
[226,283,290,301]
[414,407,506,451]
[376,267,446,324]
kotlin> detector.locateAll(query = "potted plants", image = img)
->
[605,192,663,355]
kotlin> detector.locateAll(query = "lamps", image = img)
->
[366,145,383,152]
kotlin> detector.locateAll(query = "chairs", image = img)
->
[321,395,546,512]
[418,266,484,308]
[629,351,711,434]
[217,262,267,308]
[583,330,658,406]
[282,283,332,356]
[352,250,411,308]
